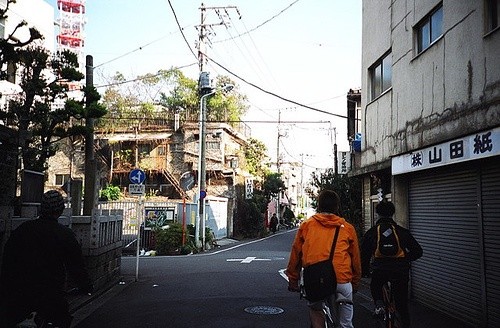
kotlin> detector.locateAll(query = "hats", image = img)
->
[37,190,65,219]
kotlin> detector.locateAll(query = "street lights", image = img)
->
[194,84,234,249]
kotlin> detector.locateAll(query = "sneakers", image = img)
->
[372,305,385,320]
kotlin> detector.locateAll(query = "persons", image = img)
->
[359,201,423,328]
[269,213,278,234]
[285,189,362,328]
[0,190,90,328]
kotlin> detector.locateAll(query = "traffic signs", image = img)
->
[128,168,148,197]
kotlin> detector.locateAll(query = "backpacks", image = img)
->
[372,221,408,263]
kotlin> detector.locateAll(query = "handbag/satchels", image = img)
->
[303,259,337,301]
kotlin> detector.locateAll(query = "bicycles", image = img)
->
[288,283,354,328]
[364,274,397,328]
